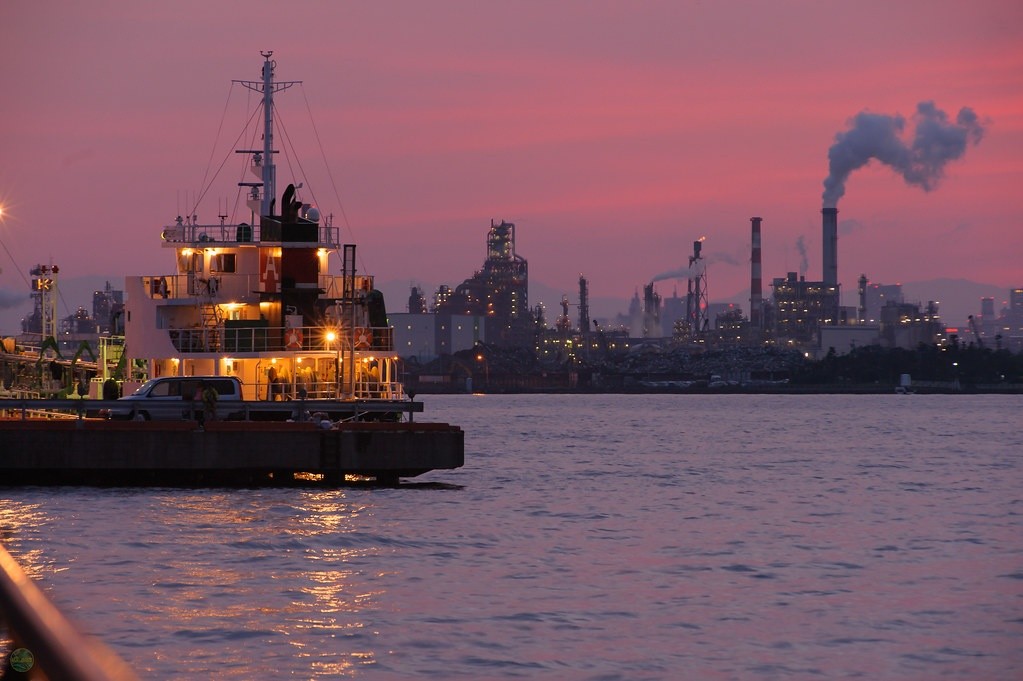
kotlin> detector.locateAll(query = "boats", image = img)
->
[120,51,403,402]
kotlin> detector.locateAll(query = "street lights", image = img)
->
[478,356,489,388]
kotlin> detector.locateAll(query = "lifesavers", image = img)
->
[203,388,219,402]
[204,409,217,421]
[353,329,373,348]
[285,328,303,348]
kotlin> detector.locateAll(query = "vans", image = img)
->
[109,376,244,421]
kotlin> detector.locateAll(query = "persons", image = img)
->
[202,384,219,420]
[269,368,285,401]
